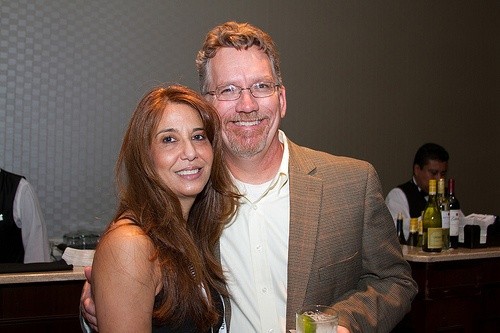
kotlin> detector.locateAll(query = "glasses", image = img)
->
[204,82,279,101]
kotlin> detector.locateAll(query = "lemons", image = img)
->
[303,314,316,333]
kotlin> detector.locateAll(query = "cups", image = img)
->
[63,231,100,250]
[295,305,339,333]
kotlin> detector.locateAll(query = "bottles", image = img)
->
[406,217,419,254]
[418,220,423,251]
[396,211,407,255]
[423,179,442,252]
[446,177,460,249]
[436,178,450,250]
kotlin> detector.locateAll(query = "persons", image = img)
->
[90,84,244,333]
[0,170,52,265]
[385,142,462,241]
[80,21,418,333]
